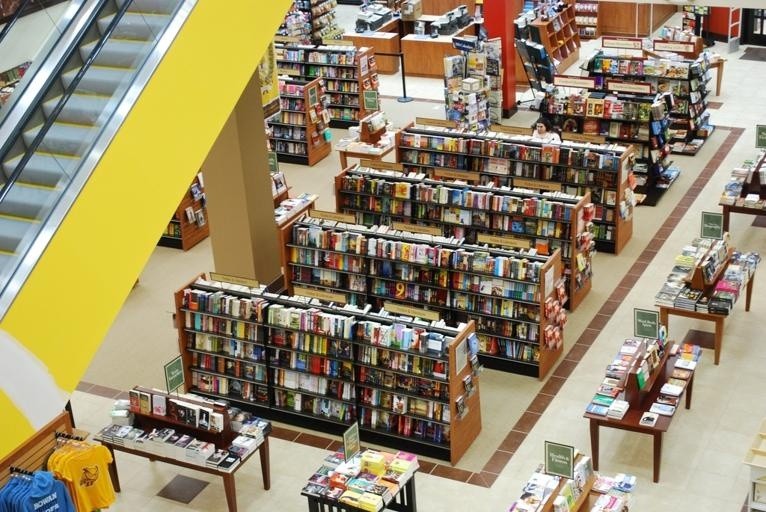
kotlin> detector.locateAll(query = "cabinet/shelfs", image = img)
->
[156,0,715,467]
[742,419,765,512]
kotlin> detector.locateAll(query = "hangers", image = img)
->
[9,432,90,482]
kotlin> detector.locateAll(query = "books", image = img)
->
[288,125,637,363]
[96,278,479,512]
[443,0,722,202]
[654,237,761,315]
[162,172,207,237]
[511,449,636,511]
[258,1,380,196]
[754,484,766,504]
[719,151,766,210]
[586,338,701,428]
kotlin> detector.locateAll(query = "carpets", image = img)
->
[739,47,766,62]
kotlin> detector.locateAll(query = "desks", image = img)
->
[719,160,766,232]
[654,238,762,365]
[509,448,633,512]
[583,338,704,483]
[91,407,272,512]
[642,26,724,96]
[301,444,421,511]
